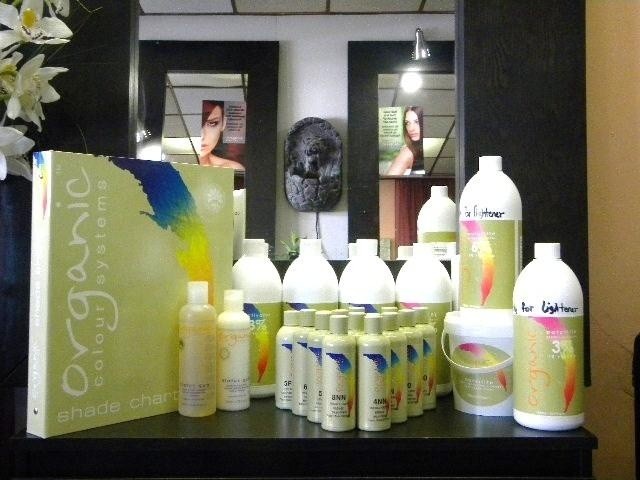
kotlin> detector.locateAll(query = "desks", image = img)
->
[1,393,600,480]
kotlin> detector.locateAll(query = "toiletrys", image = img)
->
[415,185,457,260]
[346,242,355,259]
[274,304,435,431]
[177,235,454,431]
[394,244,413,260]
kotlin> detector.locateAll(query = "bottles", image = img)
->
[321,315,356,431]
[305,309,332,425]
[231,239,265,286]
[282,239,339,325]
[339,239,396,312]
[339,242,354,281]
[282,238,305,295]
[385,312,409,425]
[291,310,317,417]
[412,306,437,411]
[312,306,399,340]
[416,186,456,243]
[356,313,393,430]
[233,242,282,401]
[274,310,301,411]
[398,308,424,418]
[396,242,454,395]
[483,155,522,275]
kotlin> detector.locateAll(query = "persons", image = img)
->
[384,105,425,176]
[200,99,244,170]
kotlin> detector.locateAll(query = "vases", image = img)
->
[0,179,30,388]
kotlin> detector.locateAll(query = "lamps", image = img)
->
[411,26,430,62]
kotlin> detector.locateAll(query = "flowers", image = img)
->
[0,0,108,186]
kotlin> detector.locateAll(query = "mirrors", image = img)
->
[161,69,251,262]
[379,63,459,264]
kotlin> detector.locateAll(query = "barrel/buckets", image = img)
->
[439,310,515,416]
